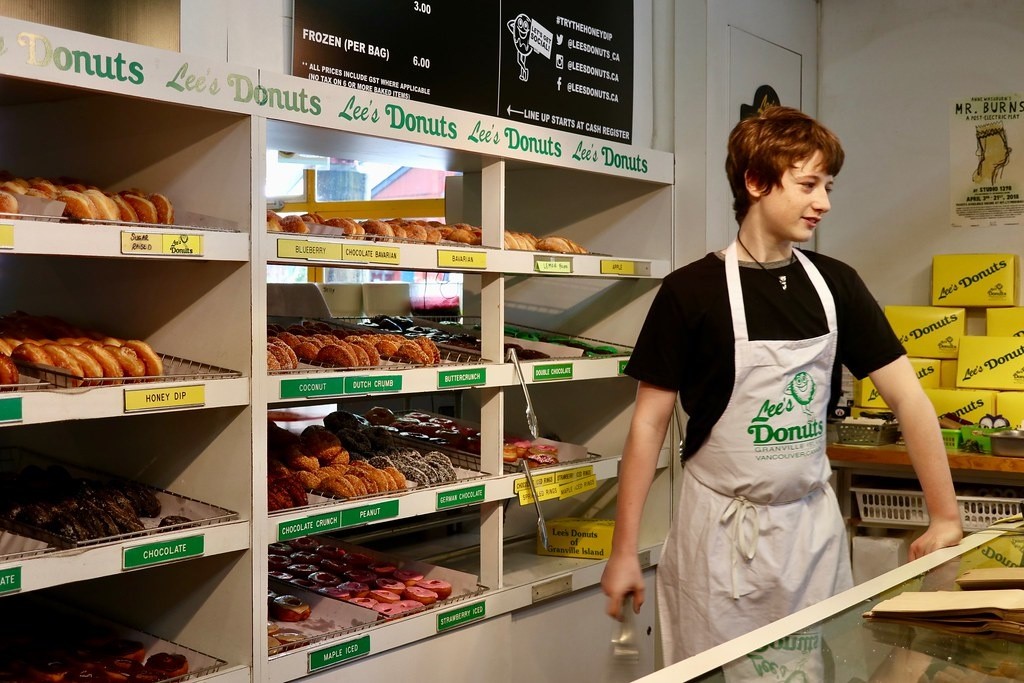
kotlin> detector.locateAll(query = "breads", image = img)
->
[265,209,591,253]
[0,169,176,227]
[909,659,1024,683]
[0,310,162,393]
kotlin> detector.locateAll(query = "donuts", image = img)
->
[0,461,198,544]
[359,315,619,360]
[266,318,441,371]
[268,404,559,511]
[268,542,451,657]
[0,615,191,683]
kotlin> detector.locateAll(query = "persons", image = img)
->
[600,109,963,683]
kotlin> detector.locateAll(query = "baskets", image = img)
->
[849,485,1023,532]
[833,422,899,446]
[940,429,961,450]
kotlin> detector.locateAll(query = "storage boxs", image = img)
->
[538,516,615,562]
[853,254,1023,432]
[852,529,1024,600]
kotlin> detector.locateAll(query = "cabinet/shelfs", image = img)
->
[1,16,678,683]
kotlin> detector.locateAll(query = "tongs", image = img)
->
[608,592,640,666]
[504,348,540,438]
[503,460,548,551]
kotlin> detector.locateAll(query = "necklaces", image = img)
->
[737,230,794,290]
